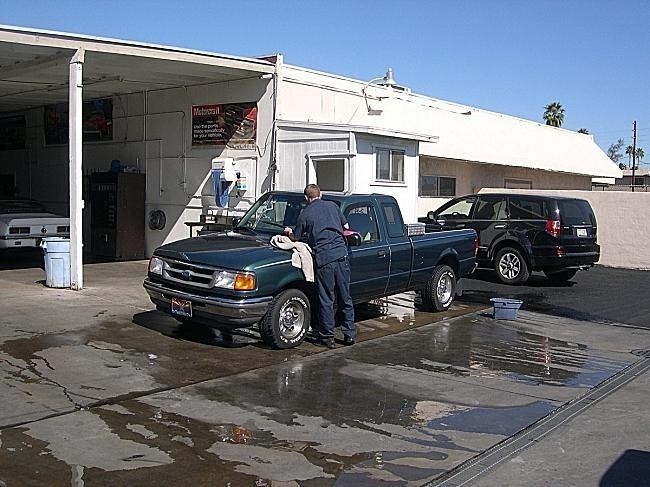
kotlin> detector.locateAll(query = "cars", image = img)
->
[0,199,69,257]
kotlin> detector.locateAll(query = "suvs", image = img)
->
[421,192,601,285]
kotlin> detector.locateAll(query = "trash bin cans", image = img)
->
[40,237,84,288]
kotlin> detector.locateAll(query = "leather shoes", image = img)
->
[311,336,336,349]
[343,336,356,346]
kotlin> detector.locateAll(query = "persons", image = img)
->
[284,184,357,349]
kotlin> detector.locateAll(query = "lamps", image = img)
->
[364,68,397,88]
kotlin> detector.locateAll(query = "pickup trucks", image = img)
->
[142,188,479,349]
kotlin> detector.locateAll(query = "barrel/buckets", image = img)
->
[41,236,70,288]
[490,297,522,322]
